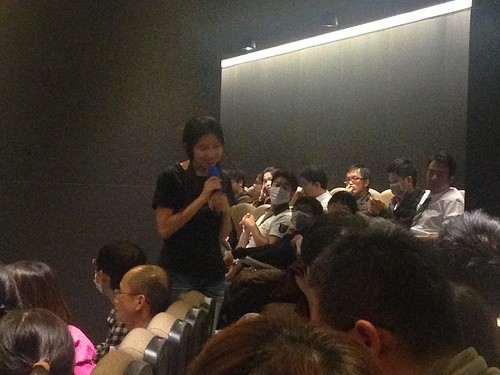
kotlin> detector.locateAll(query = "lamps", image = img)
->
[242,31,256,51]
[320,9,338,27]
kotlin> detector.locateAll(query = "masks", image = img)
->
[291,210,313,231]
[268,186,290,206]
[390,181,408,198]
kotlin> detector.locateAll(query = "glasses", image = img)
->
[92,269,101,278]
[344,177,367,185]
[113,289,143,297]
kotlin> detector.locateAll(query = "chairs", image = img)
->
[86,290,215,375]
[230,203,271,244]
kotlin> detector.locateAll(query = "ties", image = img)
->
[408,196,431,230]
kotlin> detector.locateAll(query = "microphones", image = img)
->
[208,166,222,193]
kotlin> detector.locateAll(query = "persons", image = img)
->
[0,260,97,375]
[151,116,237,330]
[187,153,499,375]
[93,240,148,364]
[112,264,170,332]
